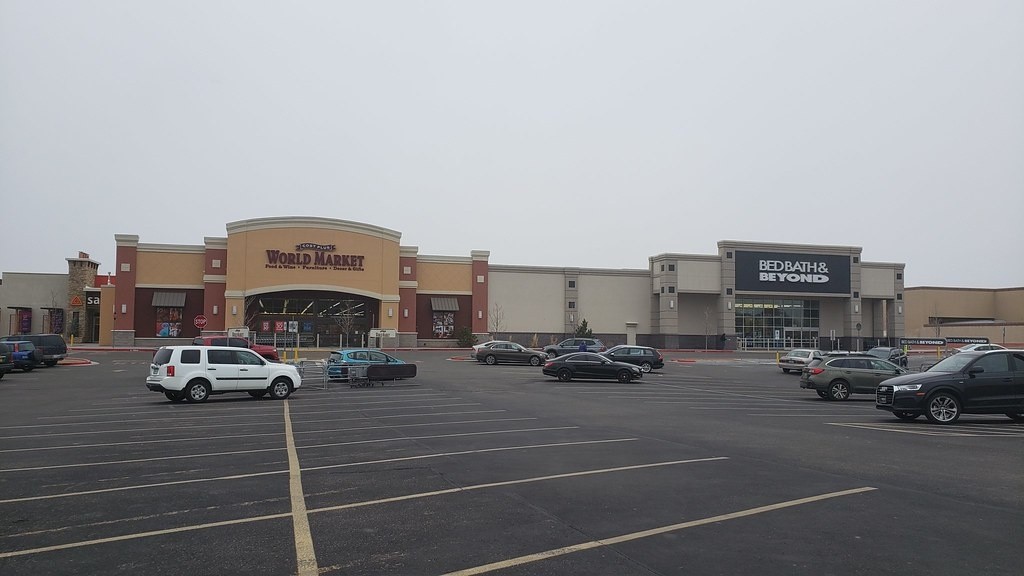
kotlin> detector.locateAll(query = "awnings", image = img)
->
[429,297,459,312]
[152,292,186,306]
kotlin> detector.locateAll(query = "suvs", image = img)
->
[144,343,304,405]
[799,351,913,403]
[598,344,664,373]
[541,337,609,360]
[876,349,1024,424]
[0,340,39,371]
[0,333,69,366]
[192,335,280,363]
[860,345,908,370]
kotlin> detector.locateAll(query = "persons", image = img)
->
[720,333,727,350]
[578,340,588,352]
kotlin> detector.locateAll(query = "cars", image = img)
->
[0,343,15,379]
[949,342,1009,358]
[470,340,548,366]
[540,352,643,384]
[326,349,406,380]
[777,347,826,375]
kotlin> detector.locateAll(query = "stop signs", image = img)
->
[193,314,208,329]
